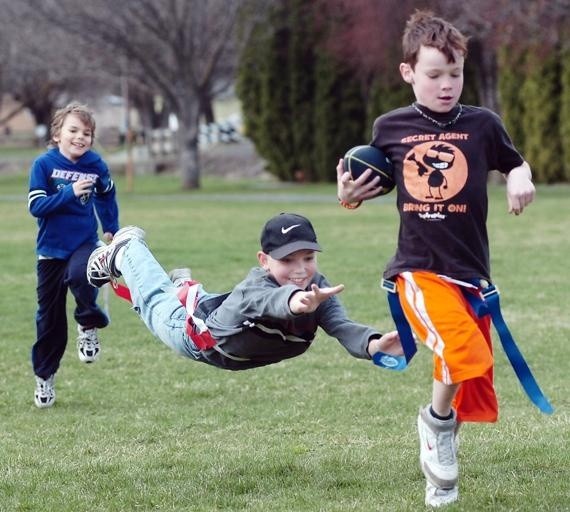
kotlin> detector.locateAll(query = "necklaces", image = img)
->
[411,100,466,129]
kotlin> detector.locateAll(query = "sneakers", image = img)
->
[169,268,192,287]
[417,402,459,490]
[425,476,460,509]
[34,374,56,409]
[86,225,147,288]
[77,323,101,364]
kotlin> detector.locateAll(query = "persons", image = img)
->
[337,7,538,508]
[31,96,121,409]
[85,211,408,371]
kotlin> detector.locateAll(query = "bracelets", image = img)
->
[341,197,358,211]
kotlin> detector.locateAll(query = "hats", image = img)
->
[260,211,323,261]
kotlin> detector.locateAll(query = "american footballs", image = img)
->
[343,144,396,198]
